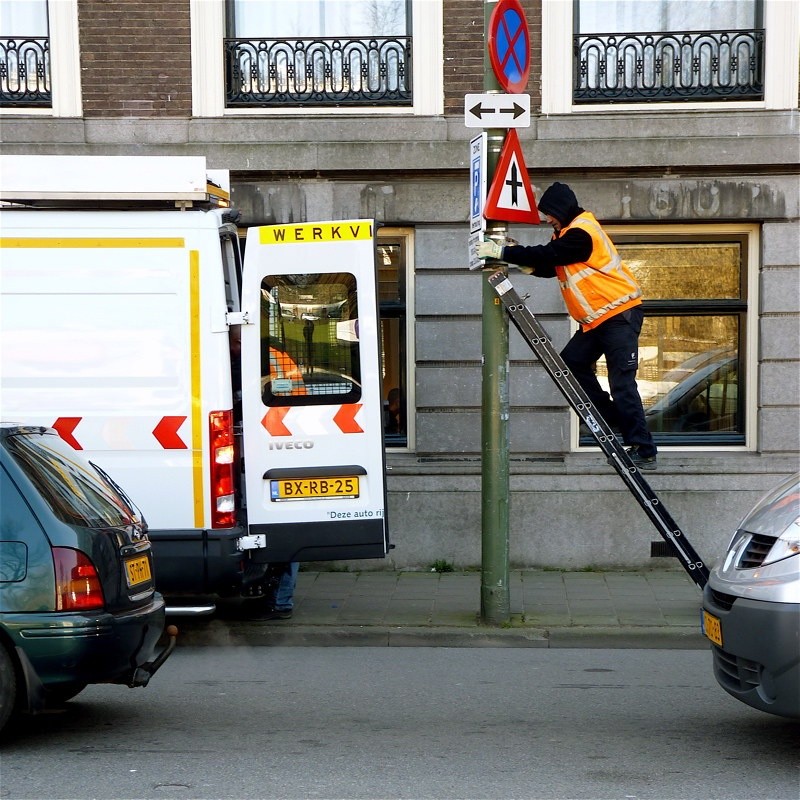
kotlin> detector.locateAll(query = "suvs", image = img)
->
[0,420,181,741]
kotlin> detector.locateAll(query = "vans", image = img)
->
[1,153,418,619]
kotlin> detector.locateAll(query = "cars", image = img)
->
[700,470,800,720]
[633,341,743,434]
[261,365,362,397]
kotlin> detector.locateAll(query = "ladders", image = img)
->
[488,271,711,591]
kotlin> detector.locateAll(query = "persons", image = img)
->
[475,183,659,470]
[229,326,306,618]
[670,395,711,432]
[382,388,401,433]
[304,319,315,375]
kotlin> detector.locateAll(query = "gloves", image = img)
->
[518,265,534,274]
[476,238,504,259]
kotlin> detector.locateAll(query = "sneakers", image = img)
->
[608,445,657,470]
